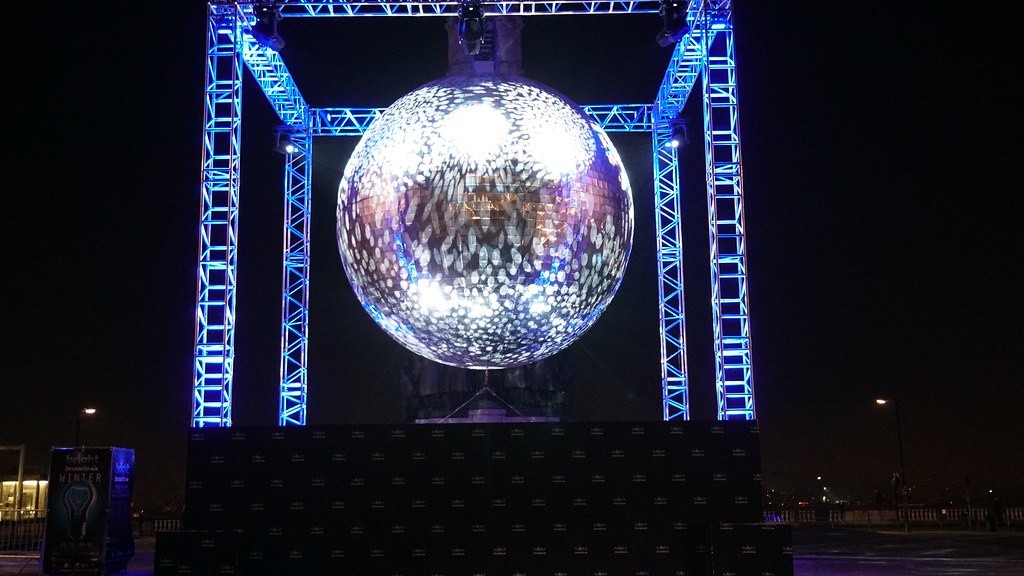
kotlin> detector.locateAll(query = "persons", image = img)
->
[874,489,884,504]
[983,487,1000,533]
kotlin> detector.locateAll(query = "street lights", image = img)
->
[876,399,909,533]
[75,408,97,444]
